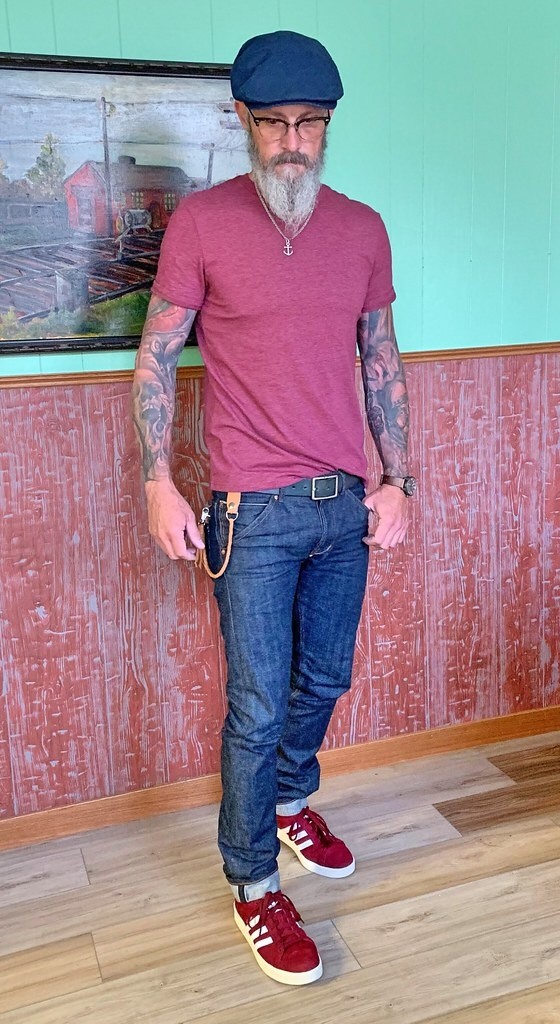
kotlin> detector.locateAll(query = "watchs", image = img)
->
[379,475,416,498]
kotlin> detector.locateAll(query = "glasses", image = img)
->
[243,101,331,141]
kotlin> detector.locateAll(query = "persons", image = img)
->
[132,31,416,985]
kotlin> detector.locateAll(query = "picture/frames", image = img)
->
[0,51,253,354]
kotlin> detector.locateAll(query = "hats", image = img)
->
[230,30,344,109]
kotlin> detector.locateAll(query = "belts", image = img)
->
[254,471,362,501]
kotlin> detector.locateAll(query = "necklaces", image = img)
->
[254,179,316,256]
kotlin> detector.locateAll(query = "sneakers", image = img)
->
[275,806,356,878]
[233,888,324,986]
